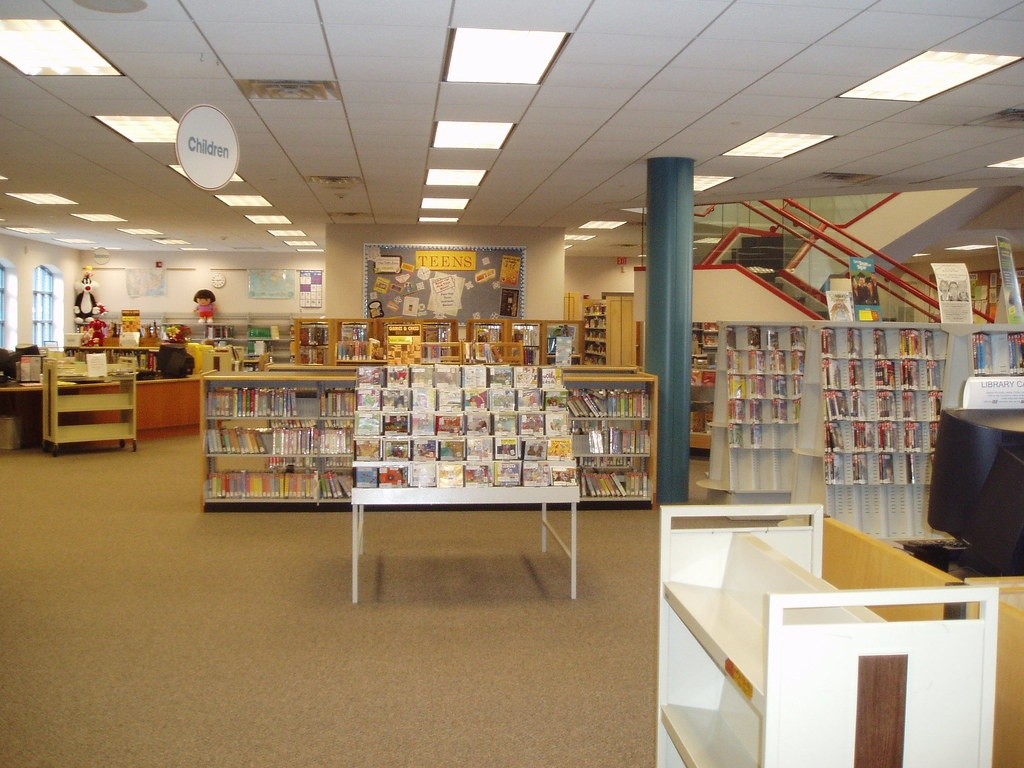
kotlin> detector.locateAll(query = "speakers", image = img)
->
[156,343,195,379]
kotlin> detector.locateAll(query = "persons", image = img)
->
[851,270,878,305]
[939,279,970,302]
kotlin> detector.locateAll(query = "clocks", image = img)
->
[211,273,225,288]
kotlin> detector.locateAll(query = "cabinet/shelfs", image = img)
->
[42,363,137,457]
[655,503,1000,768]
[63,292,1022,545]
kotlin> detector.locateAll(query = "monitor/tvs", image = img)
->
[4,343,43,378]
[927,409,1024,579]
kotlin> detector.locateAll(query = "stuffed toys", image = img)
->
[80,319,112,346]
[72,266,99,325]
[192,289,217,323]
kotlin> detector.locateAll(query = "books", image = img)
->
[567,386,653,499]
[201,324,330,370]
[206,384,355,500]
[692,321,1024,483]
[65,348,164,374]
[584,302,608,364]
[335,322,576,492]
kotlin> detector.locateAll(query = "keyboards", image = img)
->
[903,539,966,556]
